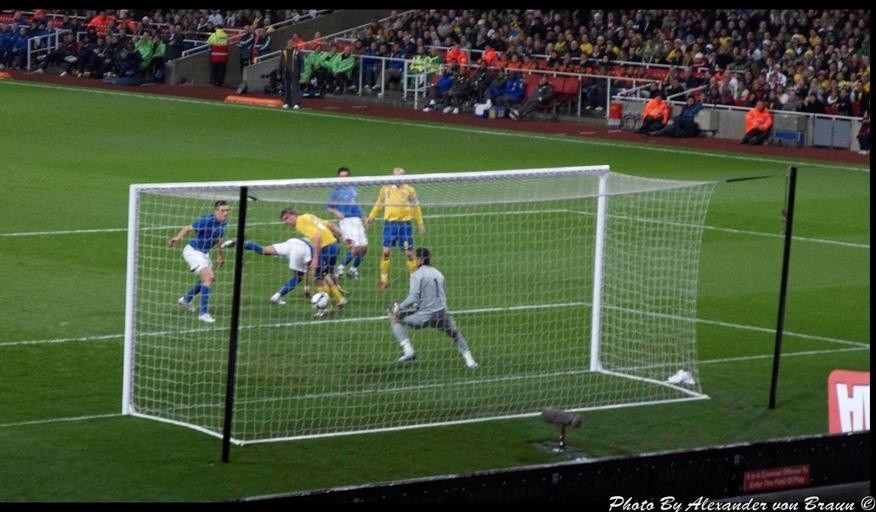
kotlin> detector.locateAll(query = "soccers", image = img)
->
[311,291,330,309]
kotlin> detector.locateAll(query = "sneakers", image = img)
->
[466,363,480,368]
[270,295,286,305]
[198,313,215,322]
[381,282,388,288]
[313,269,360,317]
[178,296,195,312]
[398,352,416,361]
[220,240,235,249]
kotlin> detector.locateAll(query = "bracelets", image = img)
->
[304,285,310,293]
[171,237,180,247]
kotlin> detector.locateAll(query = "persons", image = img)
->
[366,167,426,290]
[280,207,349,317]
[167,200,230,323]
[385,248,478,369]
[220,235,314,305]
[328,167,368,279]
[0,8,870,155]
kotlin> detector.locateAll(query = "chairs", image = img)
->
[494,58,723,99]
